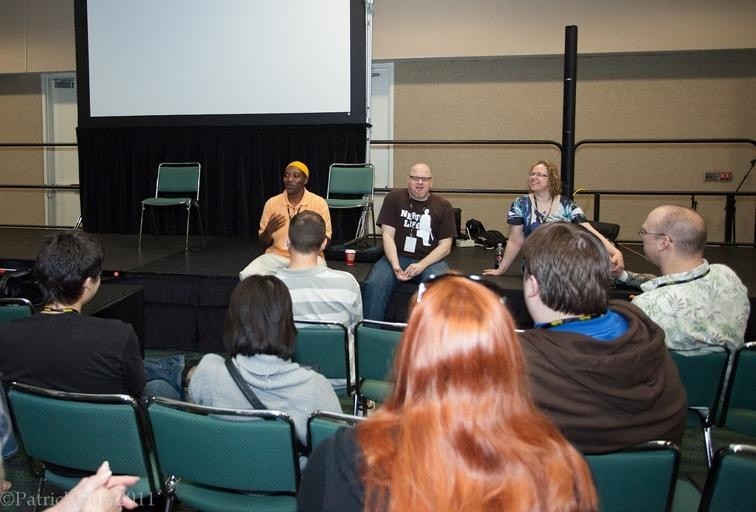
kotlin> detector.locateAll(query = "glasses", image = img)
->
[416,269,506,321]
[639,229,673,244]
[528,172,548,177]
[408,174,433,182]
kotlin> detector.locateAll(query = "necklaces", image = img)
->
[538,207,556,214]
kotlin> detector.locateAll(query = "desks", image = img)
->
[32,283,147,364]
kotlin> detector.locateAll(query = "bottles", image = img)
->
[494,243,505,270]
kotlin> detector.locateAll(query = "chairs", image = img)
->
[325,163,377,248]
[138,161,207,253]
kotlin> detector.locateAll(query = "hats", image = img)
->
[284,160,310,178]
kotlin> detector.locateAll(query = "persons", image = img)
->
[629,204,751,423]
[0,449,140,511]
[483,159,625,277]
[509,220,688,455]
[361,163,459,330]
[186,274,351,474]
[267,210,365,385]
[293,274,599,512]
[238,161,332,282]
[0,231,186,407]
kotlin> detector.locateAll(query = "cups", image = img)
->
[344,248,357,267]
